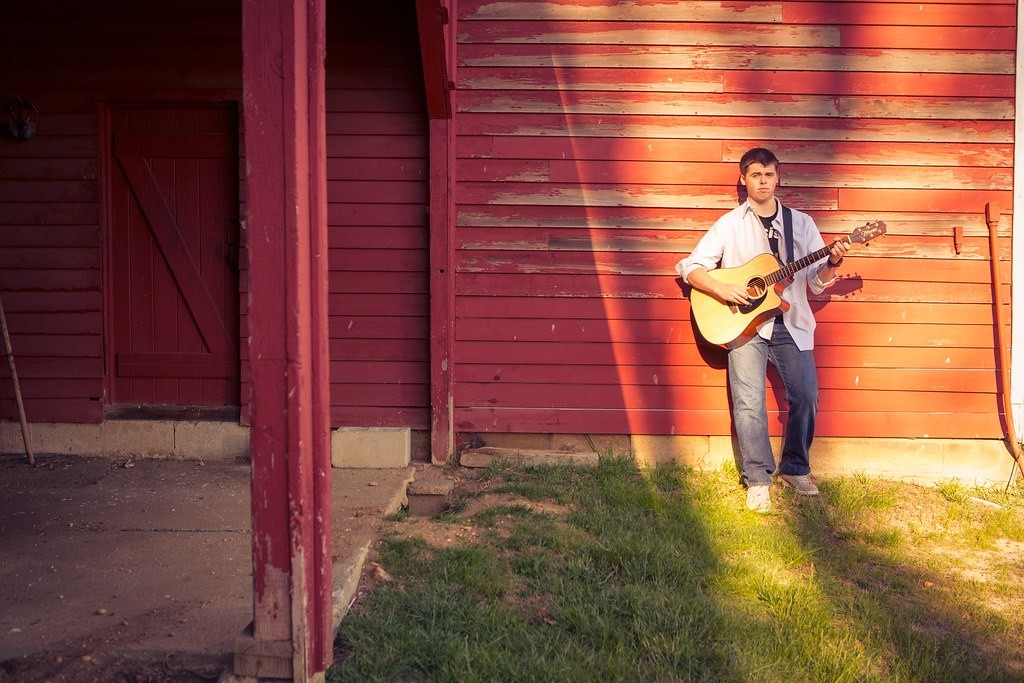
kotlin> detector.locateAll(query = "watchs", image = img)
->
[827,257,843,267]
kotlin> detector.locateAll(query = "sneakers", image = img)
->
[777,472,819,496]
[746,485,773,513]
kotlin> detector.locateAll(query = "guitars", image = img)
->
[689,218,888,352]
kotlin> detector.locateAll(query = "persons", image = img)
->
[675,147,851,513]
